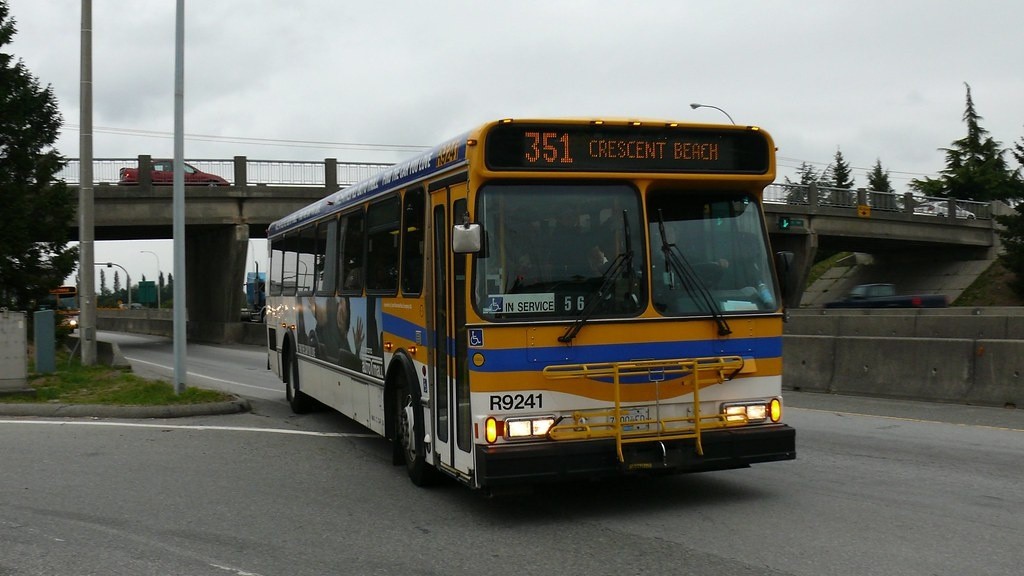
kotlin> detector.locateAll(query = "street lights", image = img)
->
[141,250,161,308]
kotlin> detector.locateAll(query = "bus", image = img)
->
[265,117,798,492]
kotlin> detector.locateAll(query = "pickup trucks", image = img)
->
[821,283,947,309]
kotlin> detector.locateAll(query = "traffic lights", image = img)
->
[780,215,790,231]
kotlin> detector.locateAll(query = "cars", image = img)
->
[59,315,79,334]
[912,200,977,222]
[118,157,231,187]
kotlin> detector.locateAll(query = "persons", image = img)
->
[582,242,614,276]
[308,292,365,368]
[634,226,730,290]
